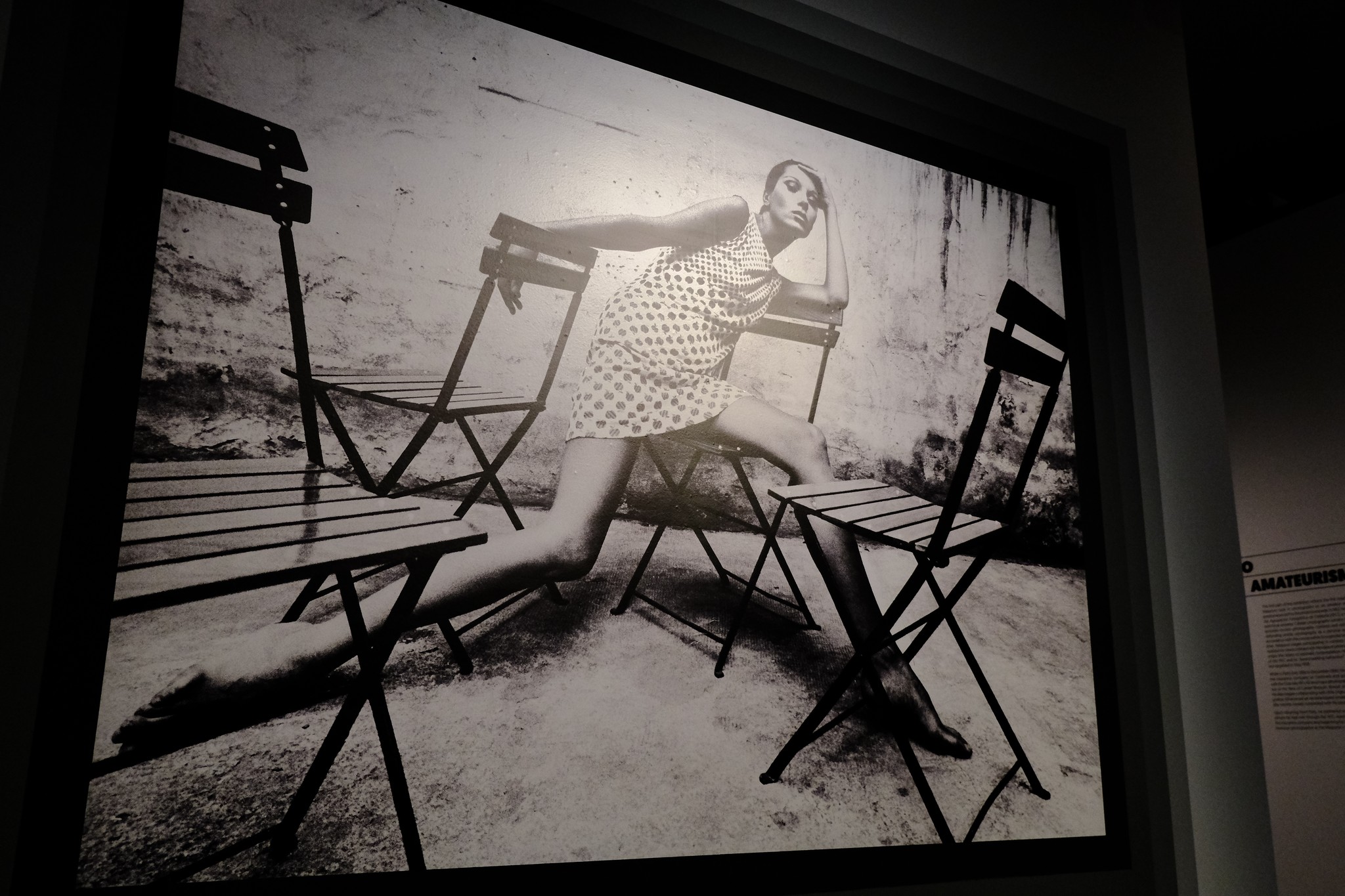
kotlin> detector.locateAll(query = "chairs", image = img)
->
[88,84,487,882]
[758,278,1077,844]
[611,293,844,679]
[273,211,599,677]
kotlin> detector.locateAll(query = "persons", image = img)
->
[110,159,972,758]
